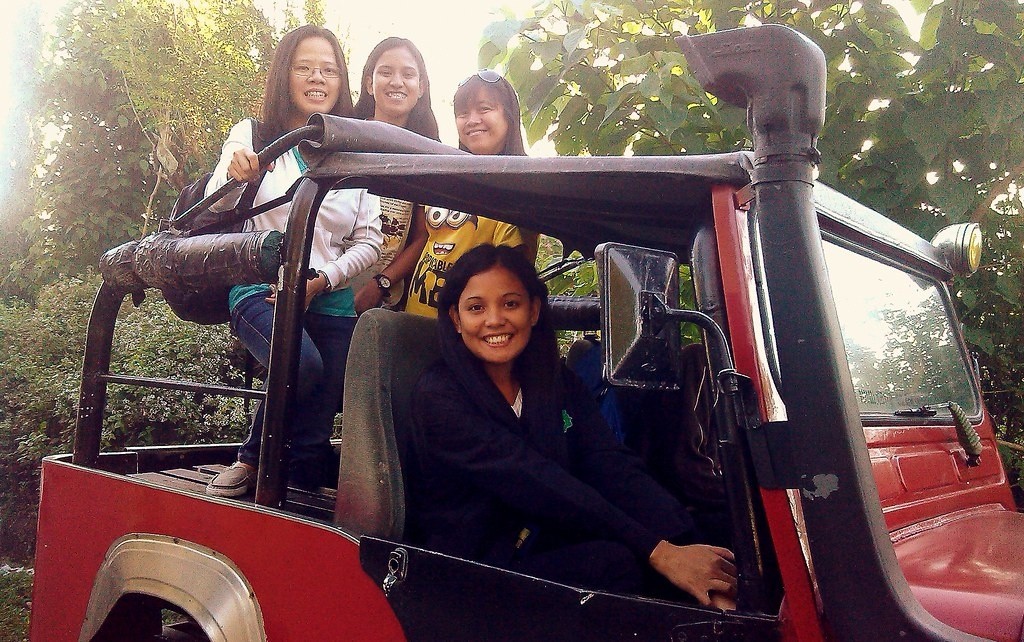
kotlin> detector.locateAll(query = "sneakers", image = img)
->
[206,459,255,499]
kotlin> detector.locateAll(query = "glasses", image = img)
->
[458,69,505,90]
[290,64,341,80]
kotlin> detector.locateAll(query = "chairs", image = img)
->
[334,307,441,543]
[567,334,629,452]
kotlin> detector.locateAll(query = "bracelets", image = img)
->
[318,270,331,292]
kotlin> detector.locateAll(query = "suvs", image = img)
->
[30,20,1024,642]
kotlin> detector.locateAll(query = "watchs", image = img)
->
[372,273,392,298]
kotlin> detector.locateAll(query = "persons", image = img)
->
[351,37,441,319]
[405,68,541,323]
[405,241,764,642]
[574,327,777,576]
[202,24,383,496]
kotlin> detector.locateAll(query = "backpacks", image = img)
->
[163,171,232,325]
